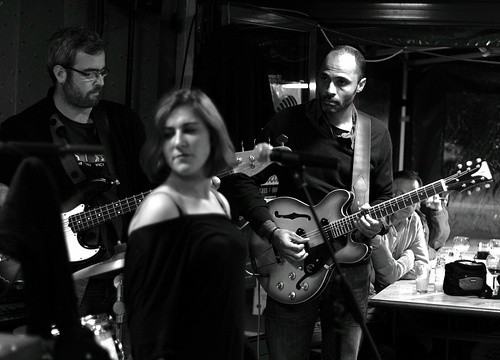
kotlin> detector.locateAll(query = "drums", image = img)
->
[81,314,124,360]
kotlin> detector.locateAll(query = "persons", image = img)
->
[370,171,450,290]
[0,26,221,360]
[121,88,247,360]
[235,45,392,360]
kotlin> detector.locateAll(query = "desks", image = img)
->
[368,239,500,360]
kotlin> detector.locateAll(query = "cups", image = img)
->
[433,246,457,292]
[487,238,500,258]
[416,264,430,293]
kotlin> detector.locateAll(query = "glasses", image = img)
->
[68,66,108,80]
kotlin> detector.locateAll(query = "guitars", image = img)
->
[61,134,293,265]
[252,157,494,305]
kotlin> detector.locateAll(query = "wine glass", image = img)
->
[453,235,470,260]
[486,254,500,296]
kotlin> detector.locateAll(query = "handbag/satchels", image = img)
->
[443,259,493,299]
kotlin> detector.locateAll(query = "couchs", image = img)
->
[447,179,500,239]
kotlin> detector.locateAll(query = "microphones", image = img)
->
[255,143,339,169]
